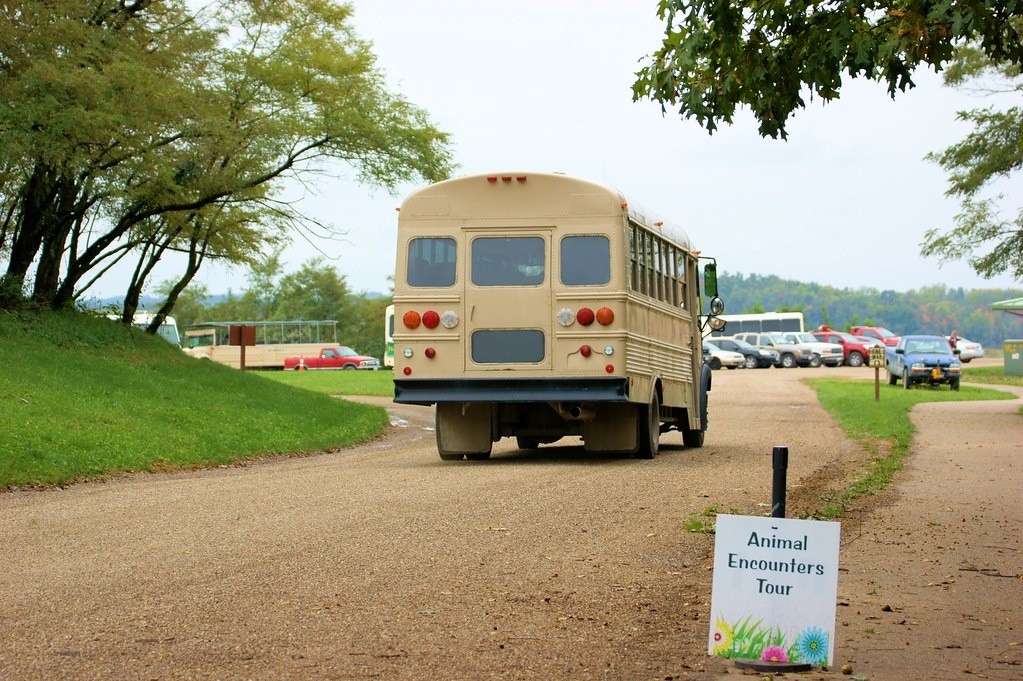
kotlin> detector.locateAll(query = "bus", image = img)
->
[181,318,341,369]
[391,171,728,460]
[382,303,395,369]
[95,312,183,352]
[701,310,805,340]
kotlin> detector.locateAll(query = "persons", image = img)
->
[949,330,959,352]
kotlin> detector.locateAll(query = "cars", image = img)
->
[708,337,780,370]
[702,340,746,370]
[945,336,985,363]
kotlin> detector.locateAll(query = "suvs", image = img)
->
[732,331,822,368]
[813,324,901,368]
[768,328,846,367]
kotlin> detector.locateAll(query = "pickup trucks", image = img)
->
[282,345,382,371]
[883,333,962,391]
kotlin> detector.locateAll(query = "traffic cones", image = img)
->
[298,354,305,371]
